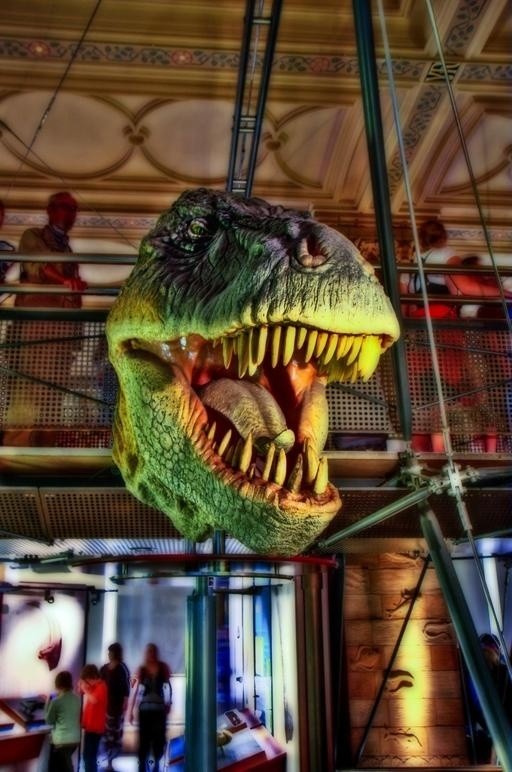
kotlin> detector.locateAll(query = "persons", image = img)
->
[75,663,110,772]
[129,640,173,772]
[42,668,83,771]
[406,215,512,453]
[2,189,88,447]
[478,633,512,728]
[95,640,133,772]
